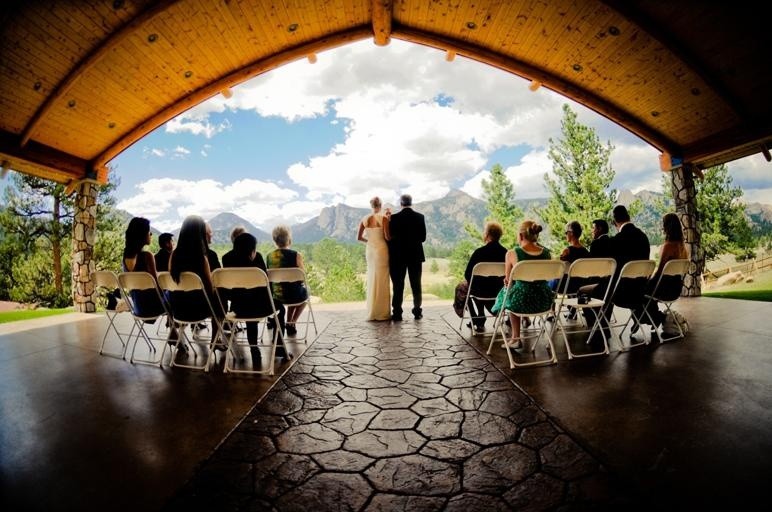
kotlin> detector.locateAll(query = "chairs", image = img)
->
[90,268,317,377]
[457,259,692,370]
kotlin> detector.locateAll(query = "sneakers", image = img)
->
[391,312,423,322]
[465,304,666,349]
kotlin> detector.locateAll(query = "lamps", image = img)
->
[529,79,541,91]
[444,49,456,62]
[222,87,234,100]
[307,52,318,65]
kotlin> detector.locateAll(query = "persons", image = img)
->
[357,197,392,322]
[464,206,690,350]
[383,207,393,218]
[389,195,427,321]
[123,214,311,359]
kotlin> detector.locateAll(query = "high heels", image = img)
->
[163,321,299,362]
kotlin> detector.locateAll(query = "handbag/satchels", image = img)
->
[662,311,690,335]
